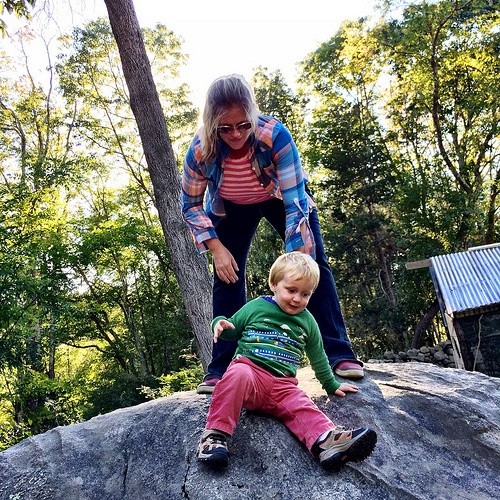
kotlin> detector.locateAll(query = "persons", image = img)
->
[180,76,364,393]
[196,251,377,475]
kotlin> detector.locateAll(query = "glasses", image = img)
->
[215,119,254,135]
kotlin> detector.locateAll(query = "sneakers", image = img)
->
[315,425,377,472]
[197,429,230,465]
[336,360,366,380]
[197,378,218,394]
